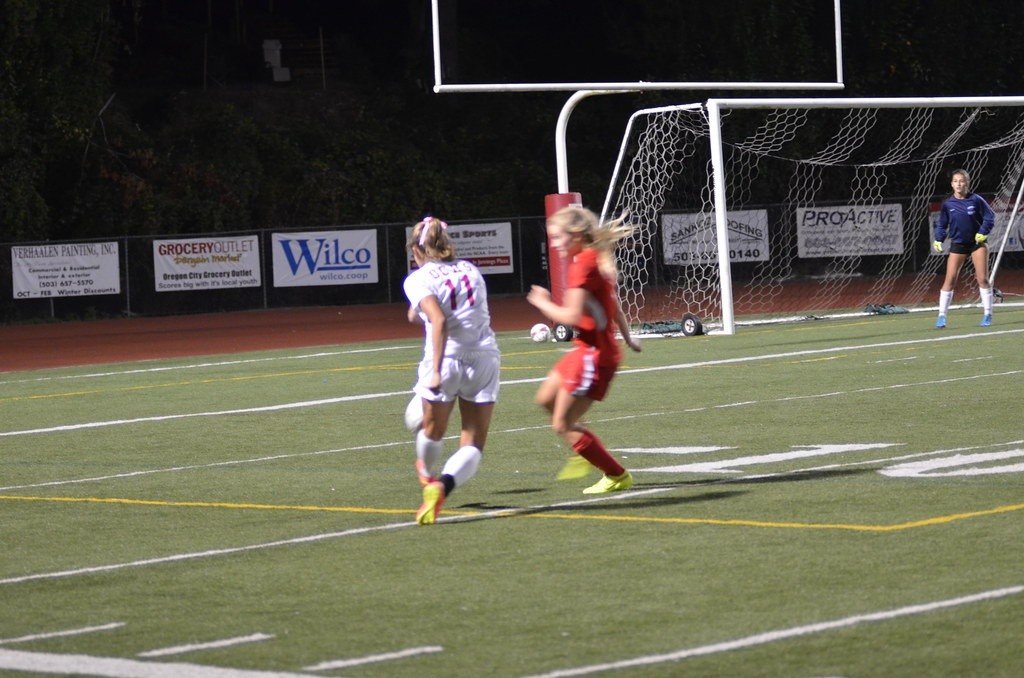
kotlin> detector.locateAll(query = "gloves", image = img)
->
[974,232,990,243]
[933,240,943,253]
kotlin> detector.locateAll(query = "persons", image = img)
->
[400,216,502,525]
[526,202,643,495]
[932,168,996,328]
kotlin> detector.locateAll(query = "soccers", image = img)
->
[405,393,426,433]
[530,322,552,344]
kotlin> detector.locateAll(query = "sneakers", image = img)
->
[937,315,946,327]
[415,481,444,526]
[583,469,633,495]
[980,313,992,326]
